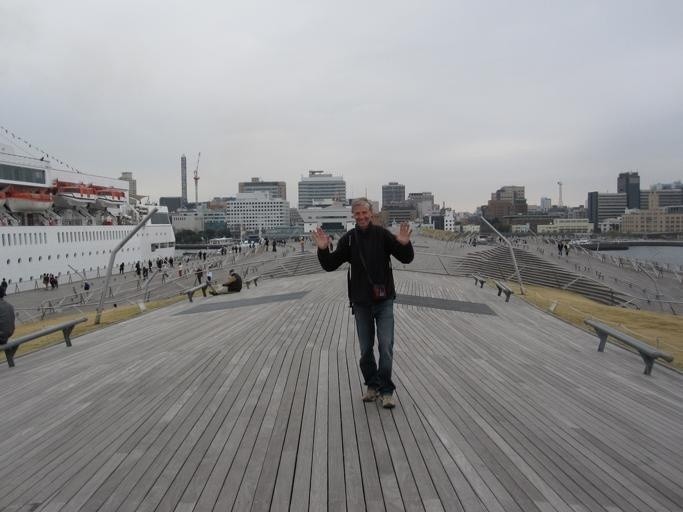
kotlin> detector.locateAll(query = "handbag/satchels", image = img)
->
[373,283,396,301]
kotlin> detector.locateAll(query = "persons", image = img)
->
[2,278,7,295]
[44,274,58,289]
[85,283,89,290]
[301,239,305,252]
[119,256,183,280]
[558,241,568,256]
[220,238,286,256]
[329,232,334,251]
[0,285,14,345]
[196,251,242,296]
[313,200,415,410]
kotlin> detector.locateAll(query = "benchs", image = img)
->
[494,280,515,302]
[472,274,486,288]
[243,276,260,289]
[180,282,210,302]
[583,318,674,375]
[0,316,88,367]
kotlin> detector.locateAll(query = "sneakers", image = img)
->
[379,394,395,408]
[361,388,379,402]
